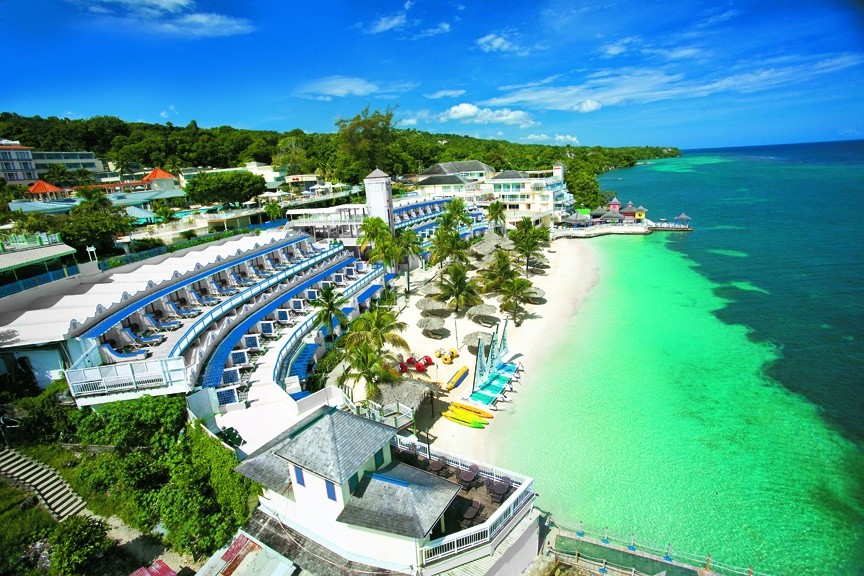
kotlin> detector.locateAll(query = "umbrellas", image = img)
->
[417,315,445,330]
[468,304,497,315]
[464,331,493,347]
[416,298,446,310]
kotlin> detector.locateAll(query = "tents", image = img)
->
[368,373,439,419]
[242,182,347,209]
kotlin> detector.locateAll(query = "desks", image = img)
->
[400,450,414,460]
[492,482,509,494]
[429,461,443,470]
[459,471,474,481]
[460,505,477,519]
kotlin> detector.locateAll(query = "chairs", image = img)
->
[454,465,482,493]
[393,444,416,457]
[479,318,493,327]
[455,499,488,529]
[529,298,540,304]
[484,476,512,506]
[427,331,442,339]
[425,456,450,476]
